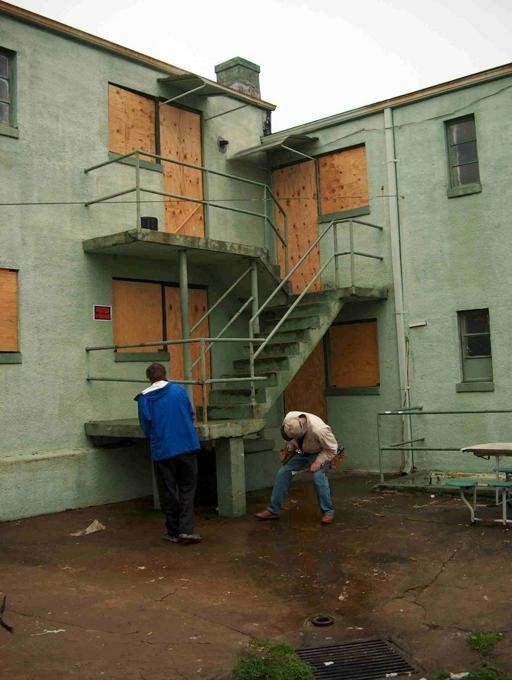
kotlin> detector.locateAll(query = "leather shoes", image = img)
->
[256,511,280,520]
[321,516,334,523]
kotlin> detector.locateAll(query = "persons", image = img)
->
[134,361,203,543]
[253,410,339,525]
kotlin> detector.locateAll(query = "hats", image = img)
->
[281,418,303,441]
[146,364,166,378]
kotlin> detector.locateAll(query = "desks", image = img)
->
[461,442,512,522]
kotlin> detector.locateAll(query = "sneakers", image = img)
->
[162,534,204,542]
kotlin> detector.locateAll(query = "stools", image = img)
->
[446,478,512,527]
